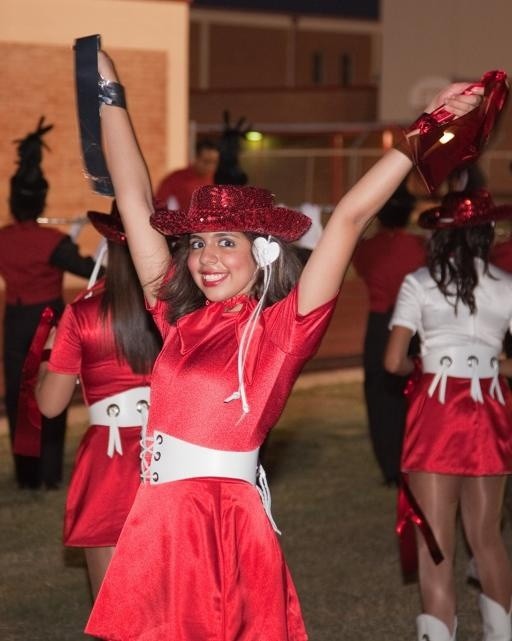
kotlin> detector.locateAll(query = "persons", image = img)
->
[157,136,228,209]
[385,190,512,641]
[0,165,106,488]
[75,36,507,640]
[34,200,164,603]
[353,187,431,487]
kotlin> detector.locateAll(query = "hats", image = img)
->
[87,198,131,248]
[149,184,313,243]
[417,189,512,231]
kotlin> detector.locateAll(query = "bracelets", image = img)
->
[395,112,445,165]
[96,80,128,109]
